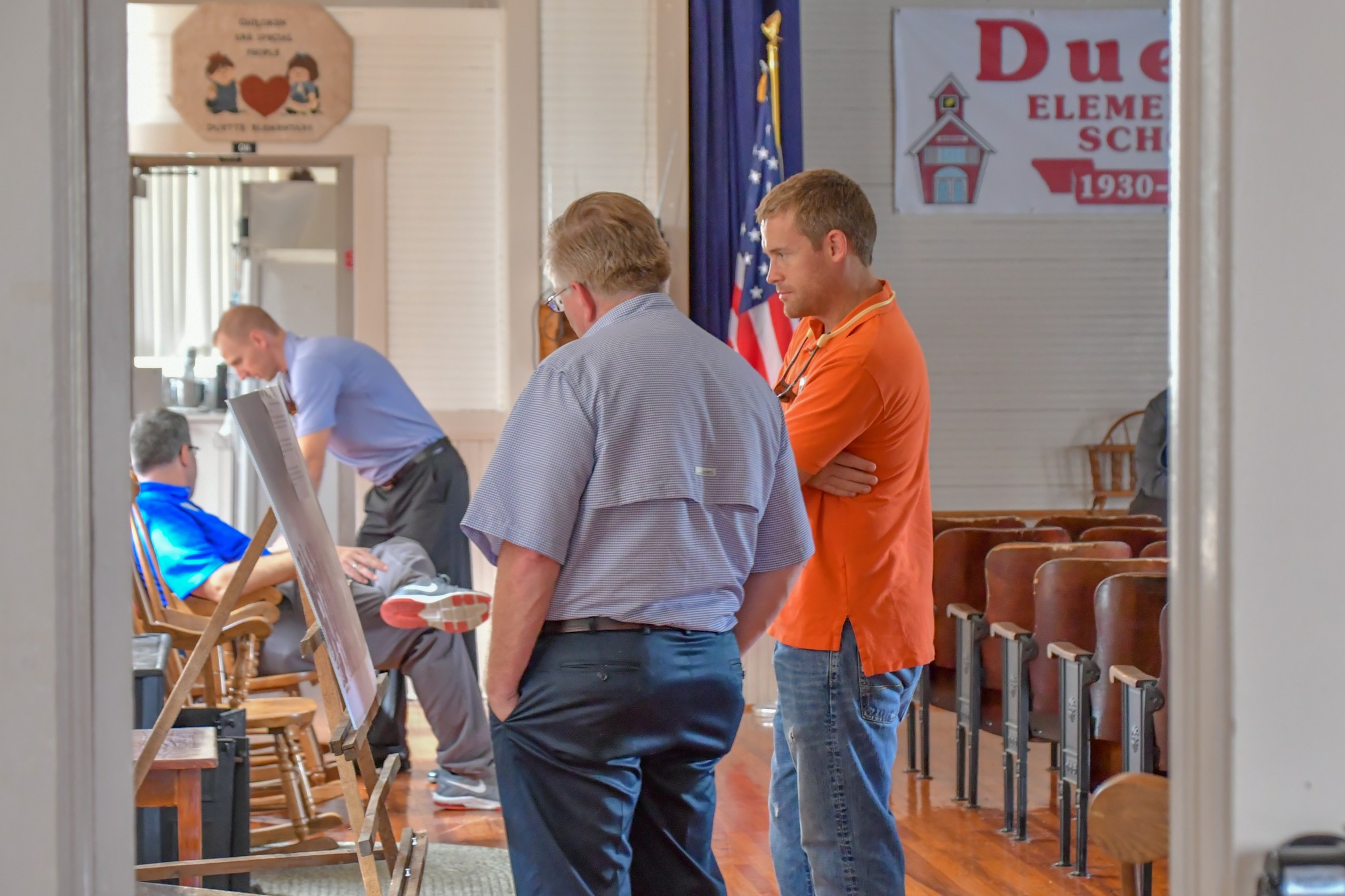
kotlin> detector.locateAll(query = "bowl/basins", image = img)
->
[753,705,776,727]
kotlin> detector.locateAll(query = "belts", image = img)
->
[540,617,675,634]
[379,436,451,491]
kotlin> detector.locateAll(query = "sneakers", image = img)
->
[378,574,493,633]
[430,768,502,811]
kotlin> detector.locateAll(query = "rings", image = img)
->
[353,562,360,570]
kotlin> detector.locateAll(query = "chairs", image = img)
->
[907,505,1171,896]
[130,485,342,859]
[169,582,350,804]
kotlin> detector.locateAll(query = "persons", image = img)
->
[456,186,819,896]
[758,166,935,896]
[210,304,478,770]
[1127,385,1170,529]
[126,409,503,812]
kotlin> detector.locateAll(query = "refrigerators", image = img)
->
[233,180,335,545]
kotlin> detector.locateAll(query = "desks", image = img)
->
[131,725,217,887]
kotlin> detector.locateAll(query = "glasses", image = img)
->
[544,284,575,313]
[179,444,200,456]
